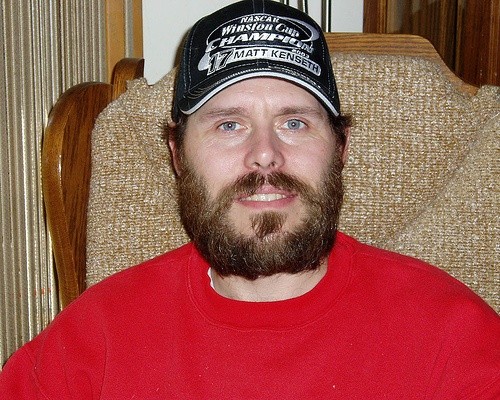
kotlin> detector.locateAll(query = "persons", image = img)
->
[0,0,500,400]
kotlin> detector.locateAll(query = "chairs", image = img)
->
[40,34,500,311]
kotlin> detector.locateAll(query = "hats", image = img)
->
[172,0,341,118]
